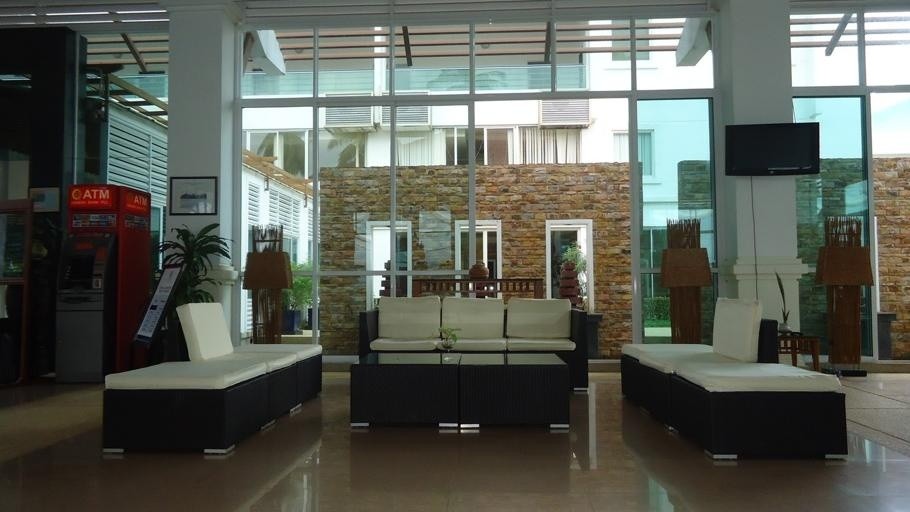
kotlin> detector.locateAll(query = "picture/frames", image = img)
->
[170,176,218,216]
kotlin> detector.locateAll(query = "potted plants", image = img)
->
[270,288,303,334]
[774,272,793,332]
[436,324,461,352]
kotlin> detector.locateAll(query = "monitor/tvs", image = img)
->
[724,122,822,176]
[66,255,93,279]
[28,186,61,215]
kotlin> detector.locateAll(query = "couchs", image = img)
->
[359,294,588,394]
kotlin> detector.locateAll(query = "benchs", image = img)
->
[102,302,321,456]
[614,295,849,463]
